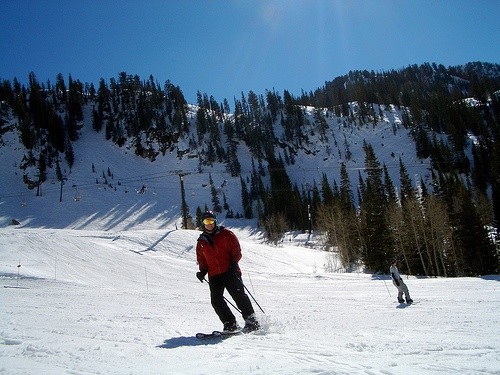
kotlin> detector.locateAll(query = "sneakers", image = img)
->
[219,315,261,334]
[398,298,414,304]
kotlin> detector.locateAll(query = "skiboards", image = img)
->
[196,327,243,340]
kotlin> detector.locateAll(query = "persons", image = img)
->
[196,210,261,332]
[389,258,413,303]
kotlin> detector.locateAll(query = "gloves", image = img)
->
[229,262,237,273]
[197,270,206,281]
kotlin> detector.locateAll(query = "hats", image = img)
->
[202,210,217,218]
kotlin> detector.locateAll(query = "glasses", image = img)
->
[203,219,214,225]
